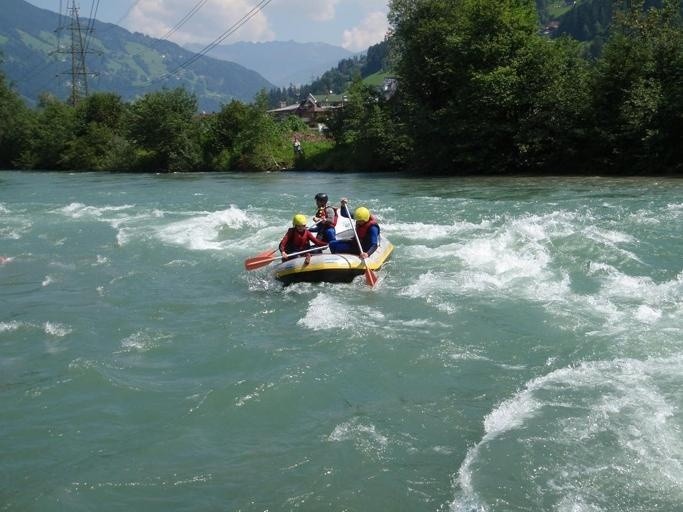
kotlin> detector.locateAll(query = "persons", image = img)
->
[329,197,380,259]
[305,193,338,242]
[279,214,329,263]
[293,137,301,155]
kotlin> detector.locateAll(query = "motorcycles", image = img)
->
[293,144,304,156]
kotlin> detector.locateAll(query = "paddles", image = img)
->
[258,220,321,256]
[341,198,378,286]
[245,246,330,269]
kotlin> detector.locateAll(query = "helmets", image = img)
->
[353,206,371,223]
[315,193,328,204]
[292,213,308,227]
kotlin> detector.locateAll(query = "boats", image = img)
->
[273,229,395,285]
